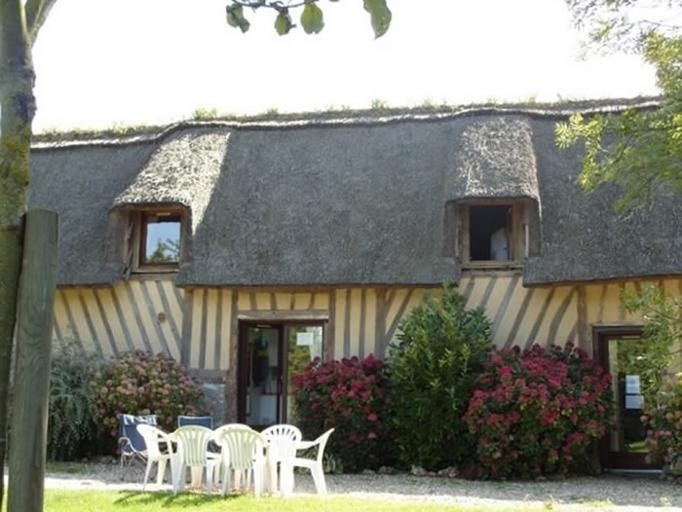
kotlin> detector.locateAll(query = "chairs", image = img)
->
[116,411,335,503]
